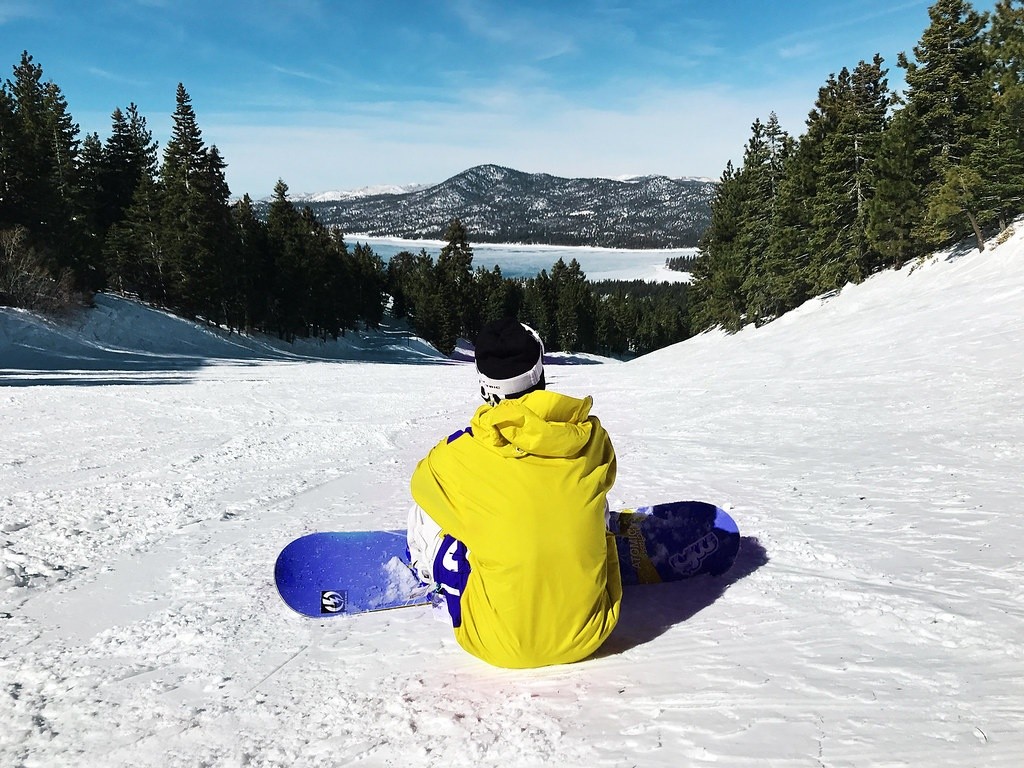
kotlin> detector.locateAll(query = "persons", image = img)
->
[406,317,622,670]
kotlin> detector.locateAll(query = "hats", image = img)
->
[475,317,545,404]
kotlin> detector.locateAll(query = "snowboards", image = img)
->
[269,500,740,621]
